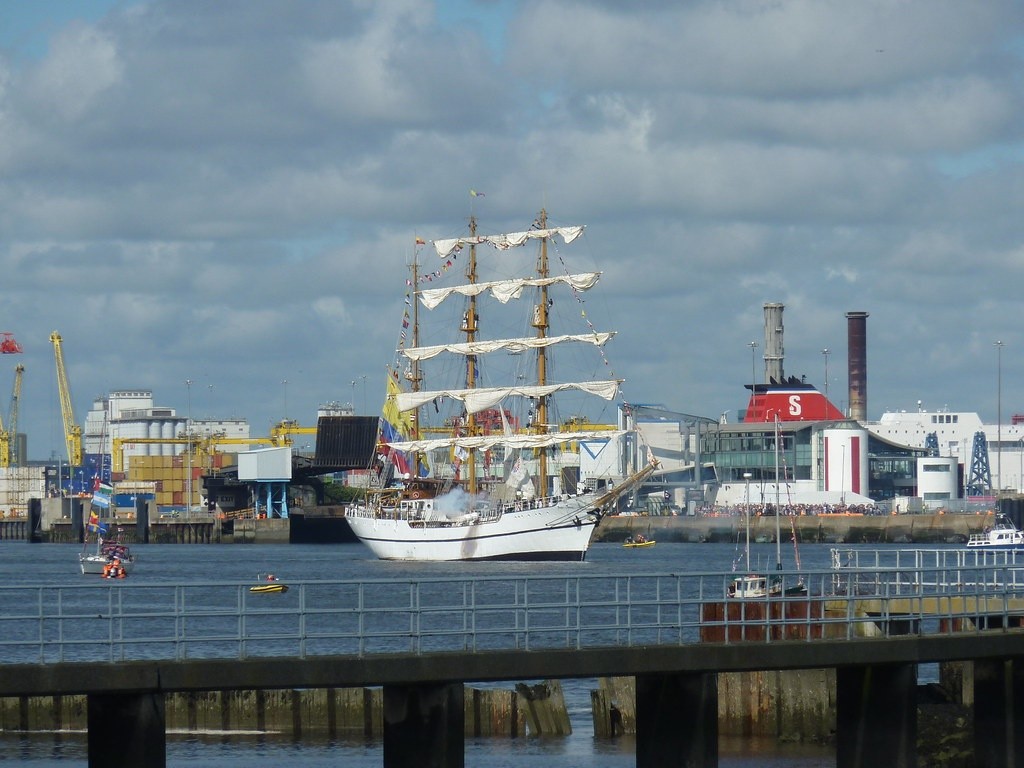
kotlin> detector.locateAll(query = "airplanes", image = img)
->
[0,332,24,356]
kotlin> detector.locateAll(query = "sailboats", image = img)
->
[74,411,137,575]
[342,187,659,561]
[719,412,810,601]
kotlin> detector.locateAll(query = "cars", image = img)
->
[609,495,888,524]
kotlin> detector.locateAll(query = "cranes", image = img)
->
[47,329,83,467]
[0,364,26,466]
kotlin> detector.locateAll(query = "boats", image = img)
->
[624,532,655,551]
[249,575,289,593]
[965,529,1024,556]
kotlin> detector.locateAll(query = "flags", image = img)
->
[390,236,468,378]
[85,476,111,537]
[469,190,486,197]
[373,370,432,480]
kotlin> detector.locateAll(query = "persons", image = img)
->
[624,533,650,544]
[495,477,614,512]
[667,502,928,516]
[526,410,534,436]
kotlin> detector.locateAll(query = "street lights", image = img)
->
[746,340,760,417]
[207,384,214,476]
[992,339,1006,496]
[361,374,367,416]
[350,378,358,416]
[280,377,288,419]
[820,347,832,420]
[184,379,195,520]
